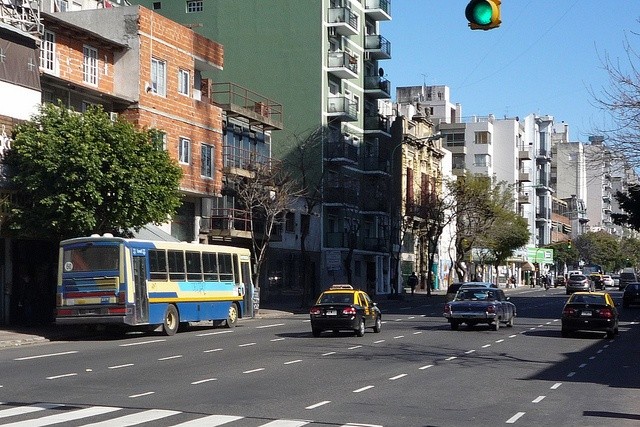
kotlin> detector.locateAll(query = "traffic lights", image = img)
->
[464,0,503,31]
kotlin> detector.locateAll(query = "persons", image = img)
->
[407,272,418,295]
[527,275,551,291]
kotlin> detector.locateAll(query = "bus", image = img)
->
[54,233,255,336]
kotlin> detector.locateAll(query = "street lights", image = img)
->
[387,131,450,253]
[508,184,543,193]
[556,210,583,241]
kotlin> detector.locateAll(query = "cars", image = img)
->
[309,284,382,337]
[460,282,498,288]
[602,275,615,286]
[566,274,596,294]
[443,288,518,331]
[622,282,640,309]
[561,291,620,338]
[589,274,606,290]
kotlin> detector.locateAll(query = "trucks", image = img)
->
[619,267,638,291]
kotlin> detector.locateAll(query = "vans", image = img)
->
[446,282,467,302]
